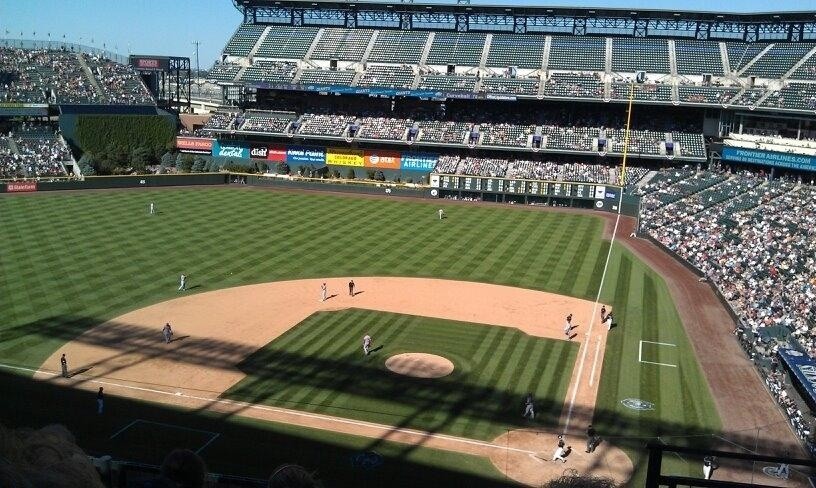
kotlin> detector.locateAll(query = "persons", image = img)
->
[607,312,614,330]
[150,202,154,214]
[322,283,327,302]
[362,333,372,354]
[178,272,187,291]
[0,42,156,184]
[564,313,574,336]
[584,424,598,454]
[182,60,640,207]
[2,387,321,488]
[348,280,355,296]
[60,353,70,378]
[162,323,173,344]
[521,392,534,419]
[438,207,445,219]
[600,304,607,323]
[641,76,816,480]
[553,433,568,464]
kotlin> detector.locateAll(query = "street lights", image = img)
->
[0,27,119,62]
[191,40,202,81]
